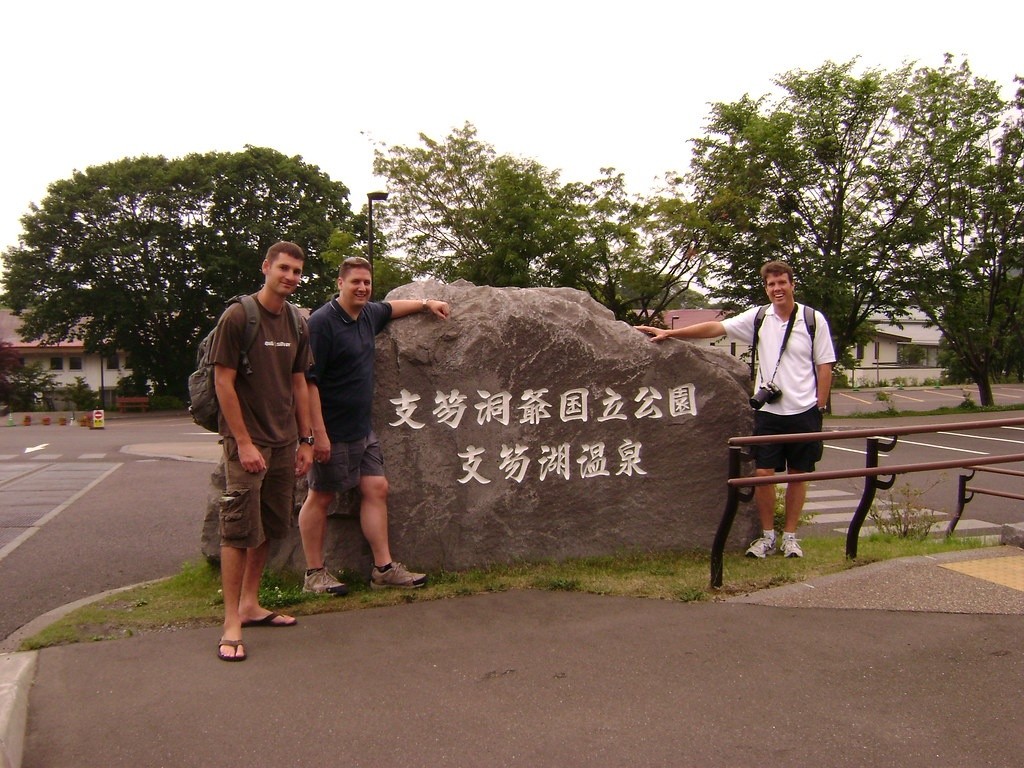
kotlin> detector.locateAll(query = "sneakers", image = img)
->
[745,529,777,558]
[303,567,348,596]
[779,532,803,559]
[370,561,427,591]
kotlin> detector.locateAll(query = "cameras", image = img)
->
[749,381,782,409]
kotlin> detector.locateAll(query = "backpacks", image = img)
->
[188,295,301,428]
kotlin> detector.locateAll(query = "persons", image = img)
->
[298,258,449,593]
[635,262,836,558]
[214,242,314,660]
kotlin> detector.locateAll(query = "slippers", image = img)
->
[242,611,298,626]
[217,635,246,660]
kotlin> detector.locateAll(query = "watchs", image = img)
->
[423,299,428,312]
[298,436,314,446]
[818,406,826,413]
